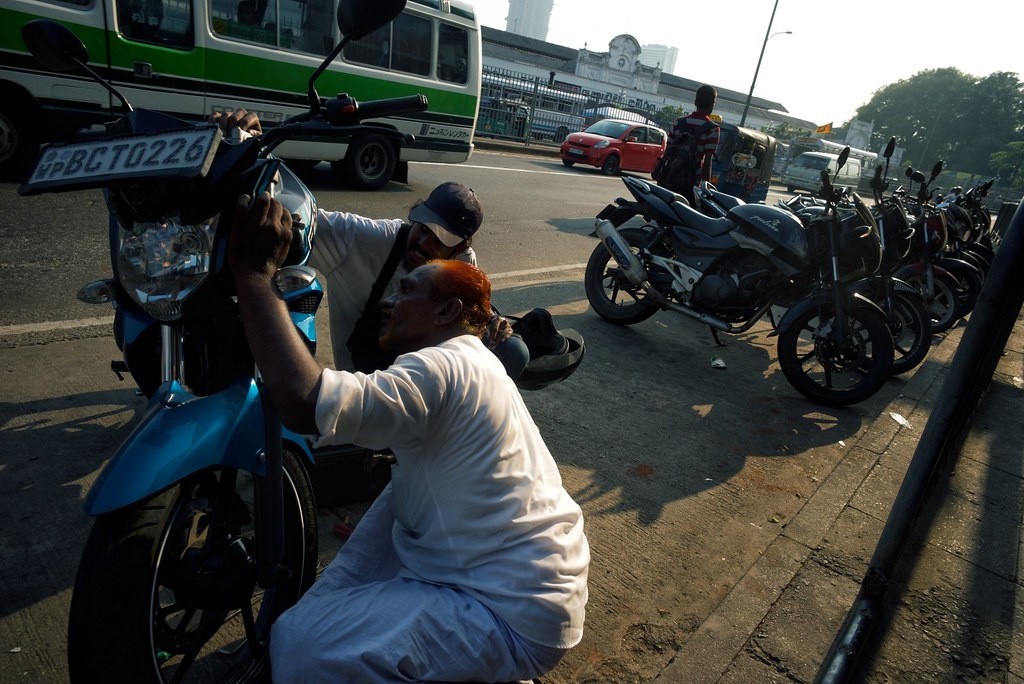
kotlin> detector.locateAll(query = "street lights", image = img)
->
[738,31,793,127]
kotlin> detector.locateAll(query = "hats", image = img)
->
[409,182,483,247]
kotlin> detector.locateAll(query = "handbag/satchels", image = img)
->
[346,305,401,375]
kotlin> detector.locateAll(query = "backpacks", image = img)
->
[657,117,720,197]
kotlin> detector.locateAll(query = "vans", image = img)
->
[780,151,862,200]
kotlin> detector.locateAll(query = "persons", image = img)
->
[227,189,591,684]
[210,106,531,391]
[651,86,721,212]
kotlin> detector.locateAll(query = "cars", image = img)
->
[558,119,667,176]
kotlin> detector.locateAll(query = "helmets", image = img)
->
[511,308,586,391]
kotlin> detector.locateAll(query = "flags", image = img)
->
[815,122,834,133]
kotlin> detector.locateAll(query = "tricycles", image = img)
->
[708,114,777,204]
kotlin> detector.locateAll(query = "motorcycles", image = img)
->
[19,0,429,684]
[584,135,999,409]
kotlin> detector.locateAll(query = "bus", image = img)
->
[0,0,484,191]
[785,137,879,193]
[474,75,588,143]
